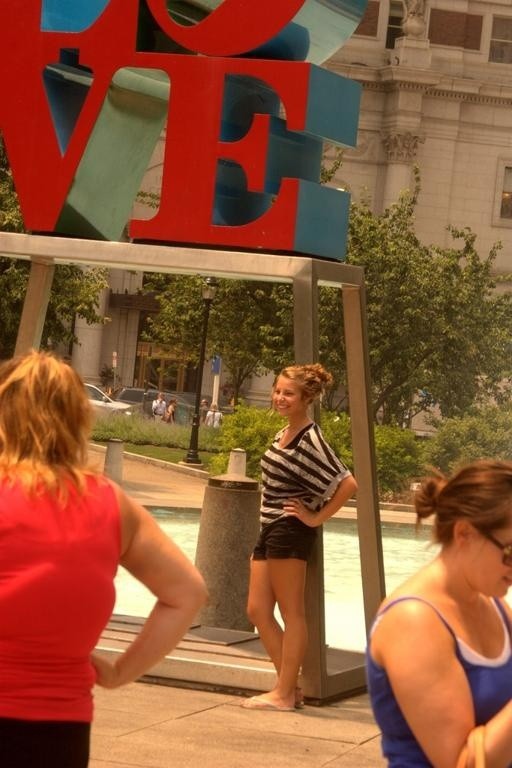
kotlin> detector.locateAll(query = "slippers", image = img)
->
[241,696,305,712]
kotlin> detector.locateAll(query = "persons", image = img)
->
[205,402,223,427]
[199,398,209,422]
[164,397,177,424]
[151,390,168,426]
[236,356,358,714]
[0,342,212,768]
[363,455,512,768]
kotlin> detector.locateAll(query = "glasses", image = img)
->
[477,527,512,567]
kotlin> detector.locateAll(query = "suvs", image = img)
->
[109,387,195,423]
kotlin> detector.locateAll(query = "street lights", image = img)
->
[182,276,220,463]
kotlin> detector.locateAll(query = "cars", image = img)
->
[82,383,133,419]
